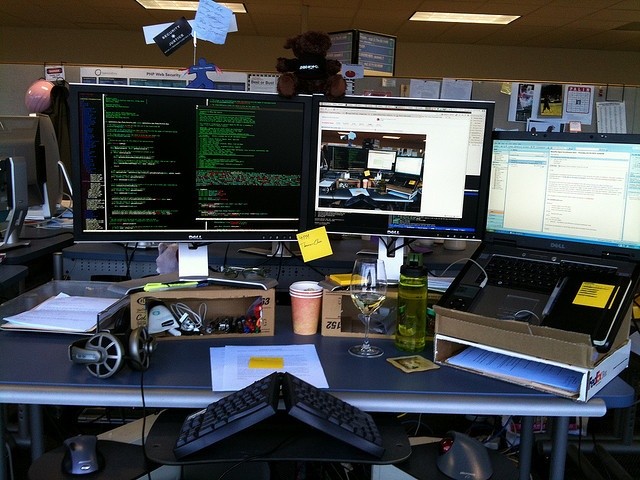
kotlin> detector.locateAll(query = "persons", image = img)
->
[401,149,410,157]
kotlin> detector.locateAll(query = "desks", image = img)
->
[1,252,635,480]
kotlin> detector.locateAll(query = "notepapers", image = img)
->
[248,356,284,369]
[330,274,368,286]
[571,282,620,309]
[191,1,232,45]
[296,226,334,263]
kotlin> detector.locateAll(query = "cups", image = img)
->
[363,179,368,188]
[397,265,428,348]
[289,282,322,336]
[380,184,386,195]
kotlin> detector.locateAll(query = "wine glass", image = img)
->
[348,257,388,360]
[373,181,379,191]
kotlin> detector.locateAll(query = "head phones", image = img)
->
[65,325,157,378]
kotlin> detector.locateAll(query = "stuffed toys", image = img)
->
[276,31,347,97]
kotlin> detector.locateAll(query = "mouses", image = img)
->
[61,433,105,475]
[435,429,495,479]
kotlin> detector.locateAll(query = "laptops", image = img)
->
[435,131,639,351]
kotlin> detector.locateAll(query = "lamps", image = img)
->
[408,11,523,25]
[136,0,247,15]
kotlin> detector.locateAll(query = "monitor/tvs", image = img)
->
[68,83,318,292]
[356,31,397,79]
[0,116,45,249]
[31,113,72,220]
[326,30,354,65]
[309,94,496,286]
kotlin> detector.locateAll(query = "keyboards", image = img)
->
[169,371,386,456]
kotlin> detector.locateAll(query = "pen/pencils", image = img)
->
[213,316,260,334]
[143,281,199,291]
